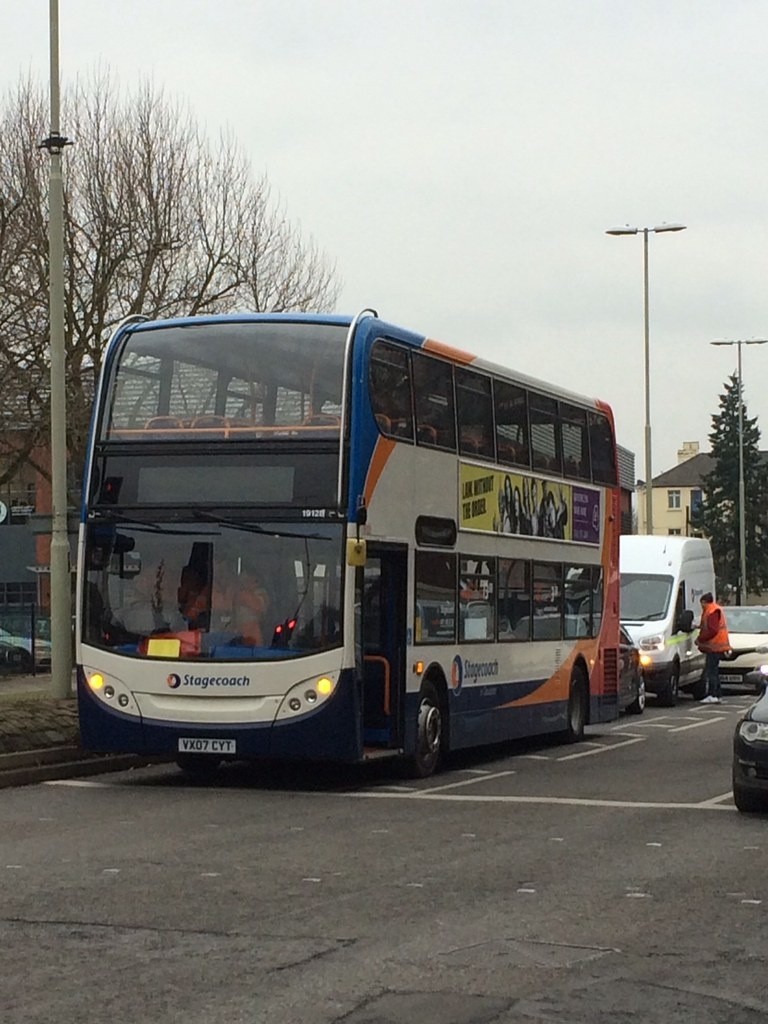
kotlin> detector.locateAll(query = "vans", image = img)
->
[717,605,768,693]
[563,534,718,706]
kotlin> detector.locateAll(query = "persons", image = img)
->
[184,553,269,648]
[492,475,568,540]
[133,544,192,602]
[691,593,732,703]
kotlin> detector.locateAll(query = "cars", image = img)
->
[0,627,53,672]
[511,613,647,714]
[731,669,768,812]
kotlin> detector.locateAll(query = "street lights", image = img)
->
[708,336,768,608]
[605,222,689,535]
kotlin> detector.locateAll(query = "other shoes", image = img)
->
[700,695,722,704]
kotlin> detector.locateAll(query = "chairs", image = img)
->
[498,444,516,462]
[726,618,736,630]
[299,415,340,435]
[533,454,549,470]
[391,419,411,439]
[739,619,749,630]
[460,434,478,454]
[225,418,257,438]
[139,416,187,439]
[375,414,392,434]
[187,416,230,439]
[417,425,436,445]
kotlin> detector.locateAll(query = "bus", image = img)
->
[71,308,623,784]
